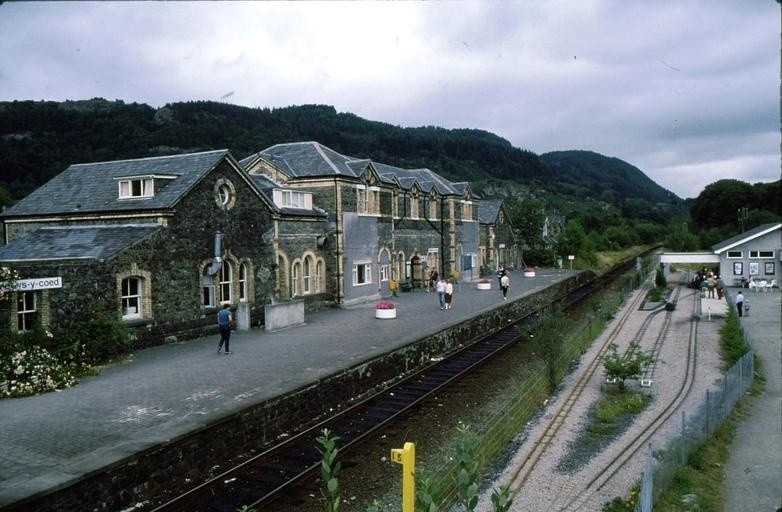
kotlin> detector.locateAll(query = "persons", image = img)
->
[495,265,505,291]
[216,304,233,354]
[500,272,510,300]
[431,266,437,292]
[423,265,432,293]
[445,279,453,310]
[735,291,744,318]
[743,300,751,317]
[692,266,723,302]
[436,278,447,310]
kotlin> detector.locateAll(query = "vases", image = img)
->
[376,309,396,318]
[524,272,536,277]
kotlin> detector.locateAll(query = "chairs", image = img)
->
[749,279,777,292]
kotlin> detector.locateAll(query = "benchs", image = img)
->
[399,282,416,292]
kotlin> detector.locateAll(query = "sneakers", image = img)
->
[440,307,443,310]
[218,346,233,354]
[425,288,429,293]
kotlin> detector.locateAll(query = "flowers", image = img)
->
[524,267,534,271]
[375,300,396,309]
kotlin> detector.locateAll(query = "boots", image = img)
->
[445,303,451,309]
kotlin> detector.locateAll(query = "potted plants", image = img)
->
[477,280,490,290]
